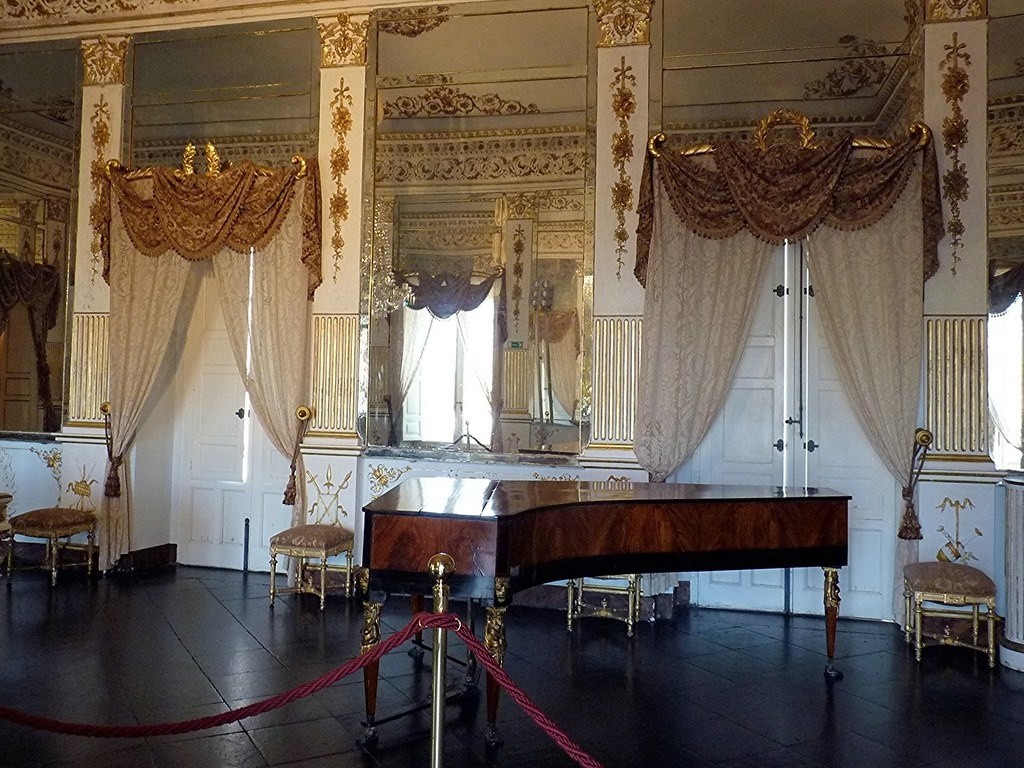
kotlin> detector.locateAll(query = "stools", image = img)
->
[902,561,998,669]
[6,508,98,589]
[268,523,354,609]
[566,572,645,636]
